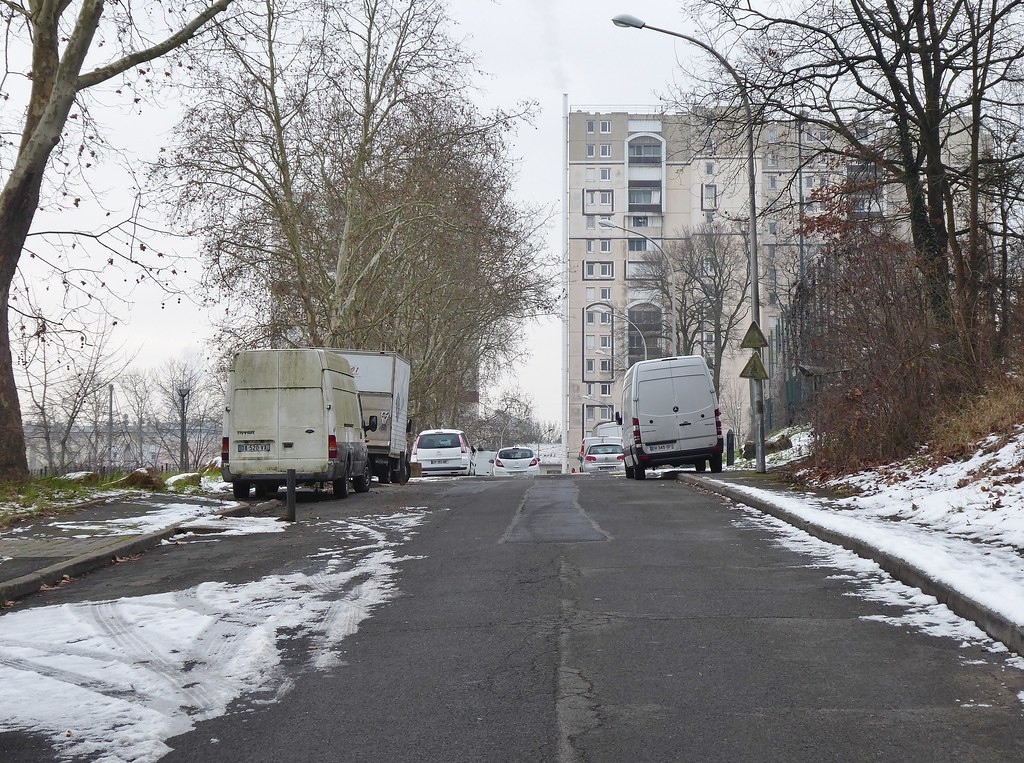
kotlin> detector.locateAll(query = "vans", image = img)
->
[614,355,724,480]
[581,437,624,449]
[591,420,622,437]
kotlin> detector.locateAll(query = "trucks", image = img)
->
[308,347,413,485]
[221,348,378,500]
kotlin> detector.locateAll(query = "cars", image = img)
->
[577,443,626,474]
[488,447,541,476]
[411,430,478,477]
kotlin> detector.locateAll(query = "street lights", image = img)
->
[175,388,197,473]
[500,416,532,448]
[537,428,553,457]
[593,308,648,361]
[597,219,680,359]
[582,396,617,421]
[609,11,779,474]
